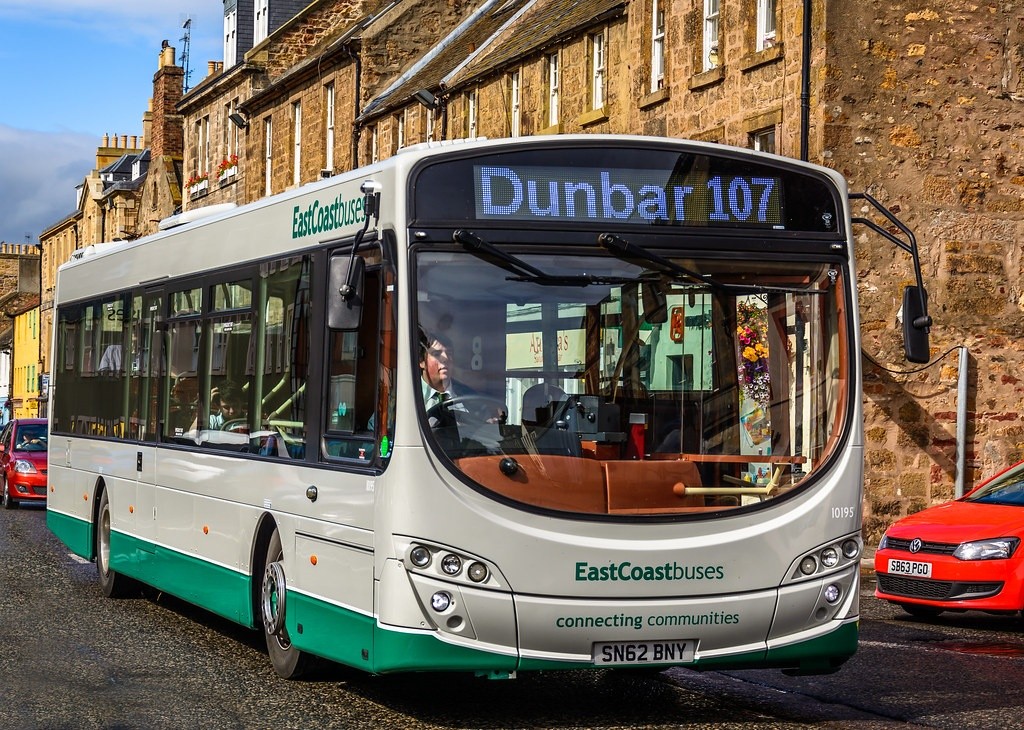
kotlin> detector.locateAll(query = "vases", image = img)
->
[189,182,200,195]
[227,165,237,178]
[197,179,209,192]
[218,169,228,184]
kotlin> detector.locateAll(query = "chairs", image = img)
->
[77,332,654,467]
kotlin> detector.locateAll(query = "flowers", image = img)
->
[195,172,209,184]
[225,153,238,170]
[215,159,230,179]
[184,176,199,189]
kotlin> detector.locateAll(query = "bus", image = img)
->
[47,135,934,687]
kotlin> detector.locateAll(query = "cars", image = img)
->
[0,417,48,508]
[873,457,1023,627]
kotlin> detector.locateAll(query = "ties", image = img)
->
[435,393,448,416]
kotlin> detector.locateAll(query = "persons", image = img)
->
[368,333,506,438]
[98,323,149,373]
[15,430,47,451]
[189,378,248,437]
